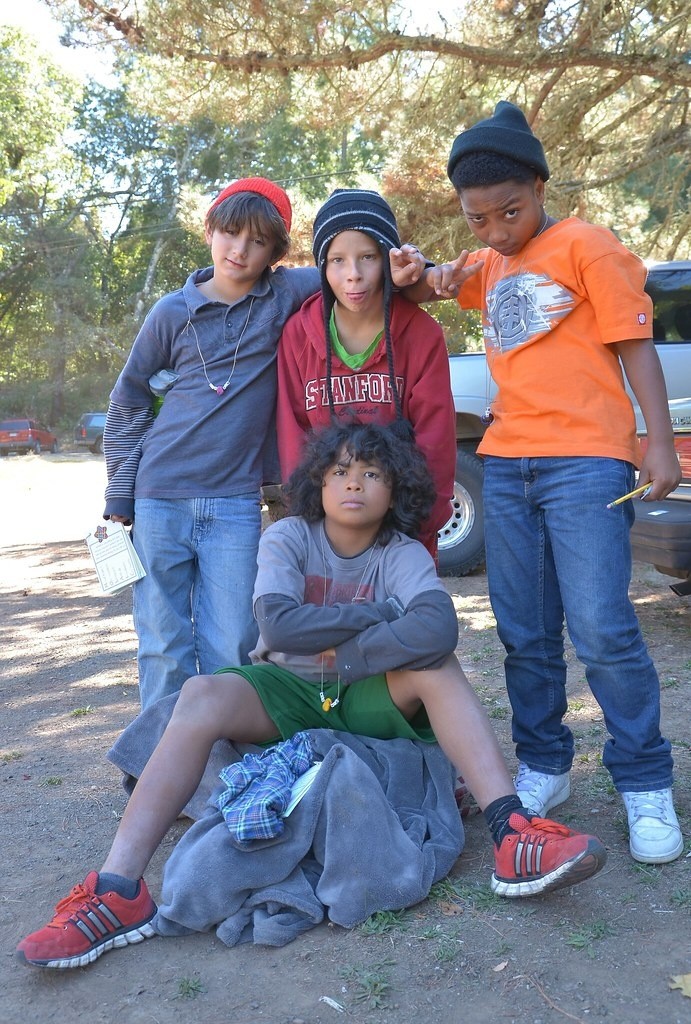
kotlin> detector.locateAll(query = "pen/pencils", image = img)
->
[606,481,652,510]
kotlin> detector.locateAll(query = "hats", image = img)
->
[447,100,549,183]
[313,188,402,426]
[206,178,291,236]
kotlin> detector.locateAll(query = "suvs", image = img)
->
[436,260,691,576]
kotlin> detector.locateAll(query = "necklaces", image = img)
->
[479,213,550,426]
[313,519,379,714]
[182,294,258,397]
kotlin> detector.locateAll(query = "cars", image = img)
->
[0,419,58,456]
[630,397,690,596]
[74,413,107,454]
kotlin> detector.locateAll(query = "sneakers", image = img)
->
[514,759,571,818]
[621,785,685,864]
[489,808,608,899]
[15,871,159,968]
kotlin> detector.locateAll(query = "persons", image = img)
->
[275,184,475,799]
[12,405,612,975]
[404,102,688,867]
[103,178,436,823]
